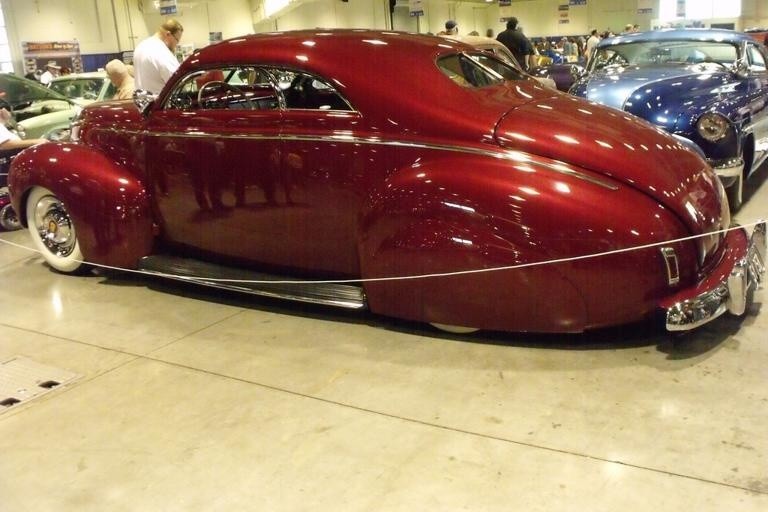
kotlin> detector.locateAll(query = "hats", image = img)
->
[45,60,62,70]
[445,21,457,29]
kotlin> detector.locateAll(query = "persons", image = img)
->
[133,19,184,95]
[445,16,640,73]
[142,128,293,216]
[126,65,135,77]
[106,59,135,100]
[0,58,76,149]
